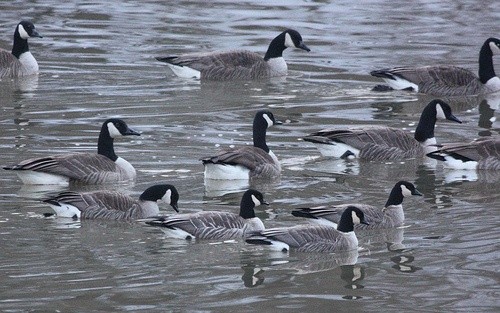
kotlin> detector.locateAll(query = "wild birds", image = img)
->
[368,37,500,97]
[200,108,284,181]
[36,183,182,222]
[0,18,44,79]
[296,96,463,162]
[290,179,424,232]
[1,117,142,186]
[154,27,311,81]
[422,138,500,171]
[143,187,271,240]
[242,204,371,254]
